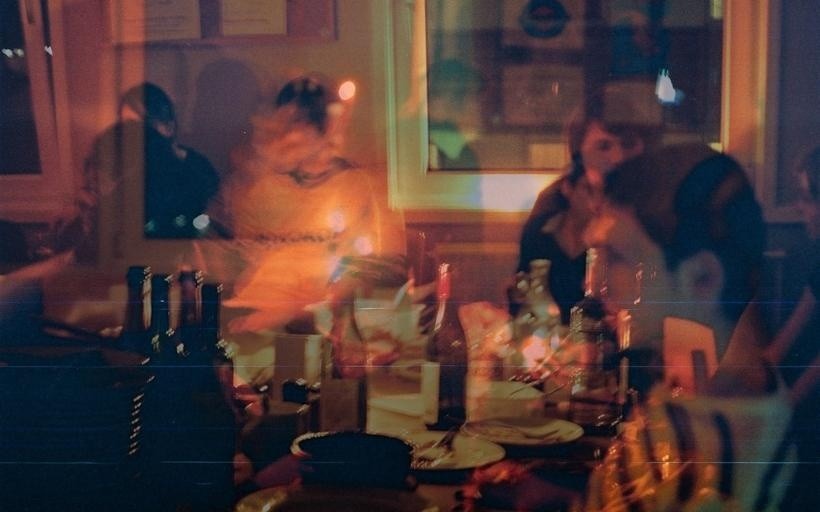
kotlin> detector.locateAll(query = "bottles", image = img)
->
[419,260,470,432]
[319,278,371,432]
[569,248,624,406]
[115,264,235,507]
[512,258,566,382]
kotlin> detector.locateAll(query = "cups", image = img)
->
[239,397,312,472]
[235,382,260,415]
[270,331,320,396]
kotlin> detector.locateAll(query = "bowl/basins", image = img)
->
[303,296,425,338]
[285,430,417,491]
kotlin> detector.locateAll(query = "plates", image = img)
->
[398,425,504,474]
[464,414,586,446]
[234,480,440,512]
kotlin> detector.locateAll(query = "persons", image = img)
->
[751,148,818,419]
[573,142,797,510]
[515,170,619,309]
[533,94,644,322]
[0,120,238,512]
[116,85,221,242]
[377,59,487,247]
[191,77,384,333]
